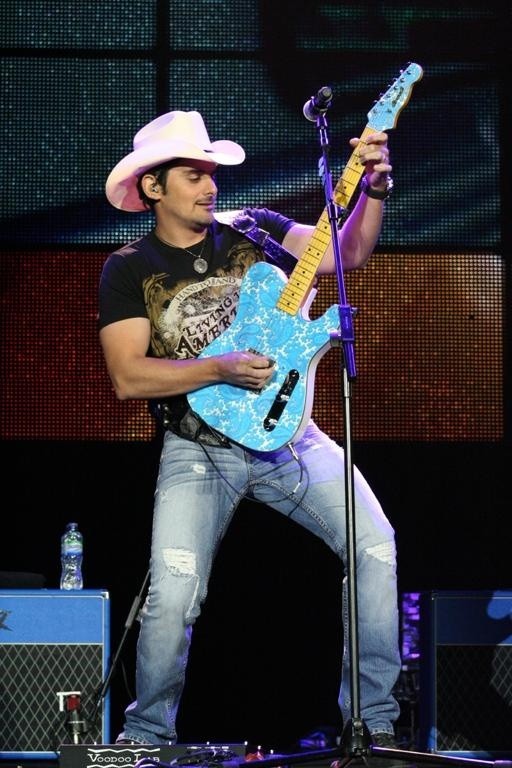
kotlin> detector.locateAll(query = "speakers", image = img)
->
[419,587,511,762]
[0,588,110,760]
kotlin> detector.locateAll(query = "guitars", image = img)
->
[186,61,423,454]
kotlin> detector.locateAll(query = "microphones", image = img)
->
[65,693,87,744]
[302,87,333,122]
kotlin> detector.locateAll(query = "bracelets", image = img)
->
[361,175,394,200]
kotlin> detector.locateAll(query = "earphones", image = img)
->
[150,187,158,193]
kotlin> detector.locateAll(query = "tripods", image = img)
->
[220,123,512,768]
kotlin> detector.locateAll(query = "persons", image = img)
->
[98,109,403,750]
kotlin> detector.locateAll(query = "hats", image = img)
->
[105,109,247,214]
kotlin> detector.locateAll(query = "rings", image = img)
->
[378,152,386,163]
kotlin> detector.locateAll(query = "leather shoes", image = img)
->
[372,731,399,751]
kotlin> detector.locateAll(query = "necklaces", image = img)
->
[154,228,208,274]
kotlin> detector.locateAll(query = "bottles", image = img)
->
[59,520,86,593]
